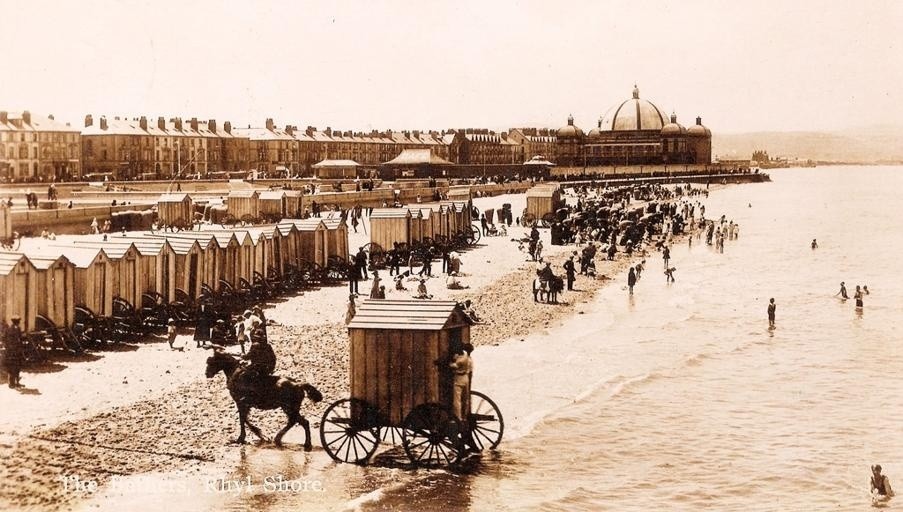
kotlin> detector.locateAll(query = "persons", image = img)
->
[46,184,51,200]
[463,342,475,414]
[51,184,57,200]
[766,298,776,325]
[41,227,48,239]
[835,281,849,299]
[1,316,25,389]
[432,344,468,420]
[853,285,863,307]
[456,303,490,325]
[863,285,869,294]
[90,216,99,234]
[166,293,267,355]
[811,238,817,248]
[239,328,276,402]
[463,299,480,321]
[175,181,181,191]
[99,219,110,232]
[868,462,893,500]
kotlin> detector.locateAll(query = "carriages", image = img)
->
[205,297,504,469]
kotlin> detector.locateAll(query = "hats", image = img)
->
[252,328,266,340]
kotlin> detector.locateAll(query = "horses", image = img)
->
[533,276,563,304]
[206,346,323,447]
